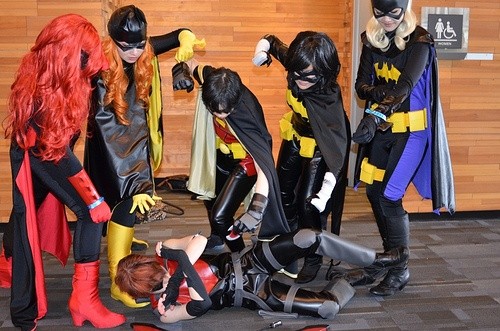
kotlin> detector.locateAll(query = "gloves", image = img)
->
[251,39,272,68]
[358,84,395,104]
[302,170,337,232]
[172,62,195,93]
[175,29,195,61]
[352,102,394,144]
[129,193,162,214]
[233,193,270,235]
[67,167,112,223]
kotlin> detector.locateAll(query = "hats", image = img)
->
[371,0,408,20]
[108,5,147,42]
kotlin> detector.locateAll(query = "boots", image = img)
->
[293,211,323,284]
[0,245,13,289]
[368,209,410,298]
[107,219,150,308]
[69,260,126,328]
[132,237,149,251]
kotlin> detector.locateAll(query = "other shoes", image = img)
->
[329,266,388,287]
[371,246,409,270]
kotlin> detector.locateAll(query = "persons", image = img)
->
[352,0,455,297]
[253,31,351,284]
[171,58,298,279]
[83,5,206,308]
[0,15,127,331]
[115,227,410,323]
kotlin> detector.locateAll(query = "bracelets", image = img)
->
[88,197,104,210]
